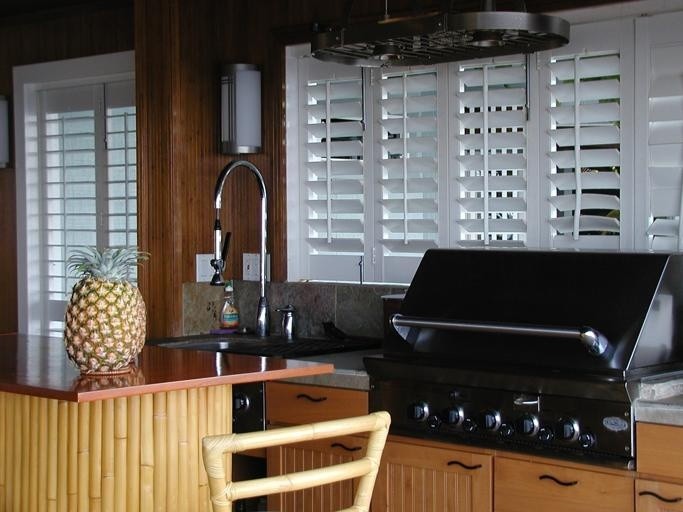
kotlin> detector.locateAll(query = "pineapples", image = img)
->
[63,244,152,374]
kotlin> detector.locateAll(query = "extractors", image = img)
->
[305,0,570,68]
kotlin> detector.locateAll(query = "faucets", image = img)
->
[209,159,272,338]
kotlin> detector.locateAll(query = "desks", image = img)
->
[0,333,336,512]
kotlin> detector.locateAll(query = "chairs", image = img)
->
[200,409,390,511]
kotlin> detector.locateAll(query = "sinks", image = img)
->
[160,337,305,359]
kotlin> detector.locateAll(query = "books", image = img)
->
[241,252,271,282]
[194,252,215,283]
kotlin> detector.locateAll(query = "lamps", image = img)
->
[219,63,263,153]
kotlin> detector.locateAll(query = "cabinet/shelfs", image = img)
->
[261,381,683,512]
